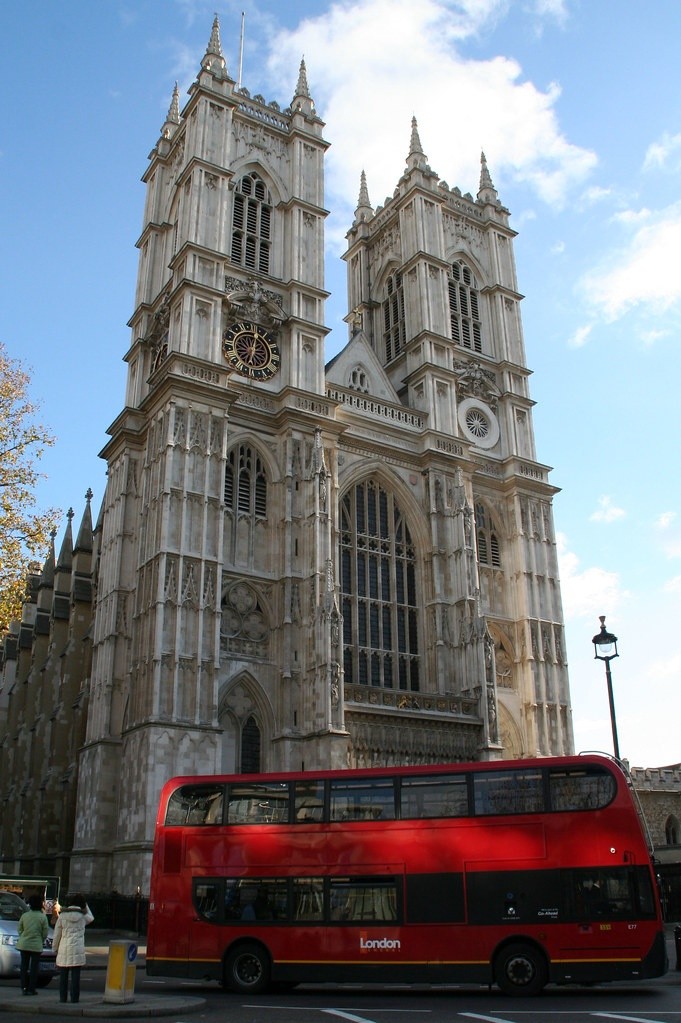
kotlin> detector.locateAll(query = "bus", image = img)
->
[146,750,669,1001]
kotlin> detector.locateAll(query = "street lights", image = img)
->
[591,616,621,770]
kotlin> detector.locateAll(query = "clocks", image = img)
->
[222,323,282,381]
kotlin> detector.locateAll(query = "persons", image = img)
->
[51,893,94,1003]
[590,878,623,914]
[52,897,60,927]
[17,895,48,996]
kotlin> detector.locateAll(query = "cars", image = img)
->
[0,890,59,989]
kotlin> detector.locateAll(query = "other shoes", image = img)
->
[28,989,38,996]
[23,987,27,995]
